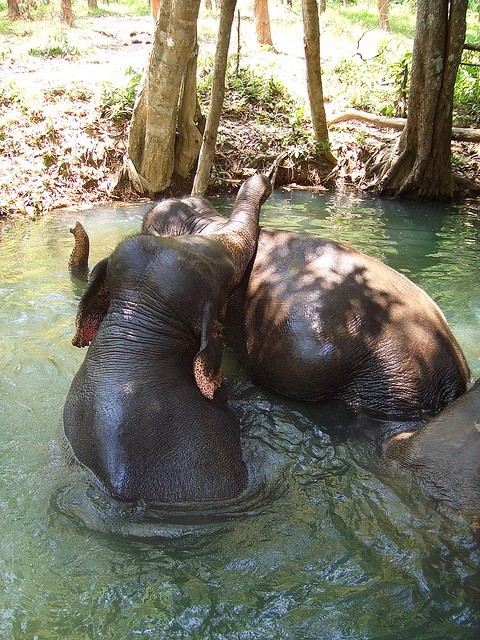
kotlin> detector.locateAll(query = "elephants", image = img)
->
[383,375,479,536]
[62,172,274,503]
[72,196,472,423]
